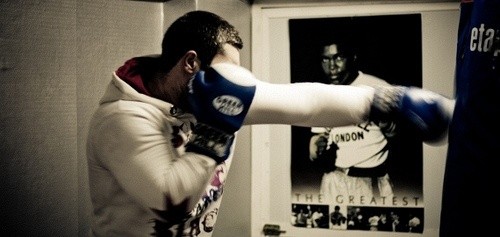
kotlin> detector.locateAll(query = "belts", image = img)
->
[330,159,389,177]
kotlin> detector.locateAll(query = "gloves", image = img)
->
[188,62,256,133]
[394,85,453,144]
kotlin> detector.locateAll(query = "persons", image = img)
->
[292,36,423,232]
[87,10,456,237]
